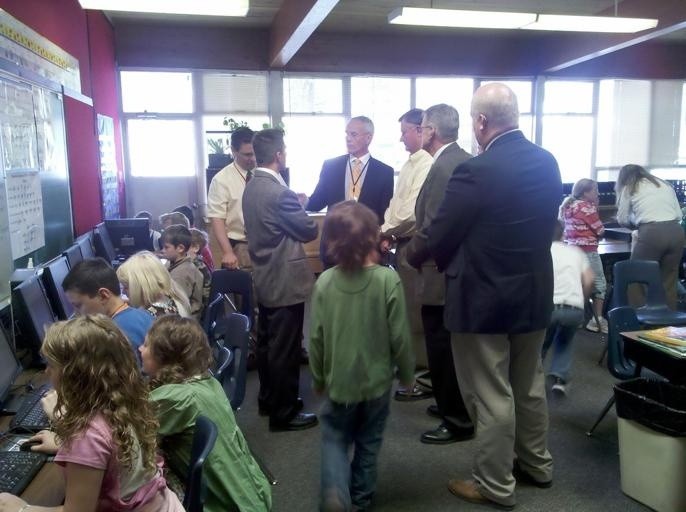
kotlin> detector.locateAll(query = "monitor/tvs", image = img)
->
[0,320,23,409]
[14,275,57,367]
[44,255,76,320]
[103,217,155,254]
[62,222,117,271]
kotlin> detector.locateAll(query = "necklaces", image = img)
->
[110,303,126,318]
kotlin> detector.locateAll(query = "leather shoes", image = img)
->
[300,347,311,365]
[513,457,552,488]
[427,406,441,416]
[449,479,514,509]
[258,396,318,430]
[395,386,430,400]
[420,424,455,443]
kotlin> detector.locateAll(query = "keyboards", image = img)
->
[0,449,49,498]
[9,382,55,433]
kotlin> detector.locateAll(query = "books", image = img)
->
[646,326,686,345]
[637,334,686,353]
[640,338,686,358]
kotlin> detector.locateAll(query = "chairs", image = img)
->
[587,306,663,435]
[598,259,685,366]
[183,268,255,511]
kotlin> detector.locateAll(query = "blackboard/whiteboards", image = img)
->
[0,69,74,270]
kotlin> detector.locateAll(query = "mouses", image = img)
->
[19,440,44,452]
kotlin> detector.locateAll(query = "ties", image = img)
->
[246,171,253,183]
[348,159,362,198]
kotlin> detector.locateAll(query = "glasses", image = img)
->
[418,126,431,133]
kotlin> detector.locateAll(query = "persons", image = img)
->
[308,200,417,512]
[41,315,273,512]
[161,225,204,316]
[136,211,161,249]
[205,127,310,369]
[558,178,609,333]
[295,117,394,272]
[405,104,475,445]
[381,109,434,400]
[241,130,319,432]
[63,258,157,354]
[117,250,190,320]
[541,219,595,395]
[1,312,187,512]
[428,82,564,507]
[613,164,686,312]
[156,206,215,286]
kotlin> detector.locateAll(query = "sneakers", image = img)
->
[552,377,565,396]
[588,315,608,334]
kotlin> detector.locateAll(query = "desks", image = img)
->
[620,329,686,380]
[598,238,630,282]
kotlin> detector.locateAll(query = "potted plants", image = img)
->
[207,137,231,167]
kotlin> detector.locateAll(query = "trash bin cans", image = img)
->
[614,376,686,512]
